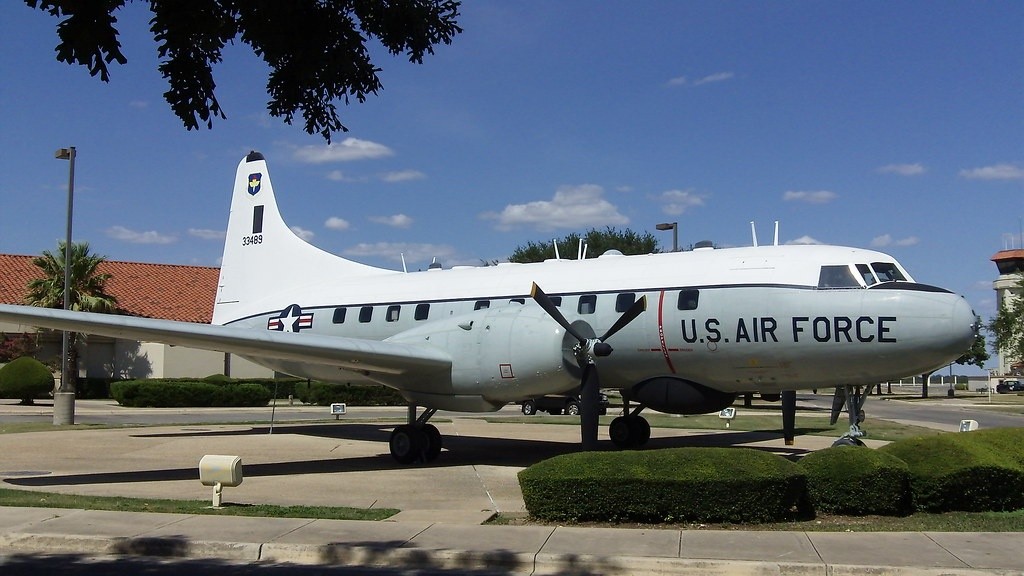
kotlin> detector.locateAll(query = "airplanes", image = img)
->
[0,151,983,466]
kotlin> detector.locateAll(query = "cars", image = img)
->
[521,390,609,416]
[996,380,1024,394]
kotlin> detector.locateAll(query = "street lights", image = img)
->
[54,146,77,431]
[655,222,678,251]
